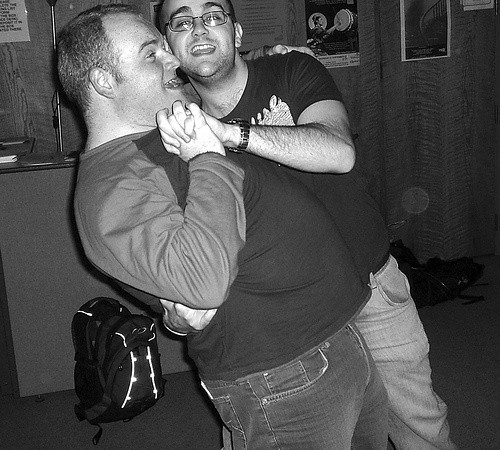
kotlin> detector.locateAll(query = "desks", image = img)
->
[1,136,196,403]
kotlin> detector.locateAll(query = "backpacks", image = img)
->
[71,298,166,423]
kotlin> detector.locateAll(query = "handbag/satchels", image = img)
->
[423,257,475,294]
[390,241,426,303]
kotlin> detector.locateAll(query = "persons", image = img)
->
[309,13,339,57]
[55,3,390,450]
[156,0,457,450]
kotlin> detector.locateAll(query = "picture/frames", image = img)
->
[399,0,451,63]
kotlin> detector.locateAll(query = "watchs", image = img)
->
[226,117,250,153]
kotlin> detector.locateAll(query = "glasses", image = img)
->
[164,10,234,37]
